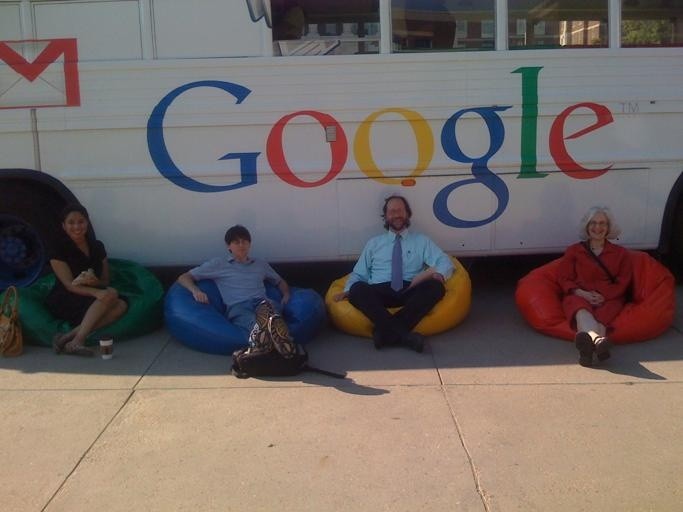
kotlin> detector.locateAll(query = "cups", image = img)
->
[100,333,113,360]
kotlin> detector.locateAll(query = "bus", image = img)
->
[0,2,682,286]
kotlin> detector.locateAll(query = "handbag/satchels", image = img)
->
[0,313,24,358]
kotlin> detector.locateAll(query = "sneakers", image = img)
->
[372,328,389,348]
[64,343,94,357]
[52,332,64,355]
[407,332,425,353]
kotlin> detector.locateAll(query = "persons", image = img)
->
[336,194,458,352]
[554,202,634,366]
[44,203,128,355]
[176,223,304,375]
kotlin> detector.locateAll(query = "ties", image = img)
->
[391,234,404,292]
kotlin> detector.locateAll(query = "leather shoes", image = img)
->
[593,336,609,361]
[575,332,594,367]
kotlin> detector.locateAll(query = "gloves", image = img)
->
[71,267,101,290]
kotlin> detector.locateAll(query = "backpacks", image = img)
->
[230,343,308,379]
